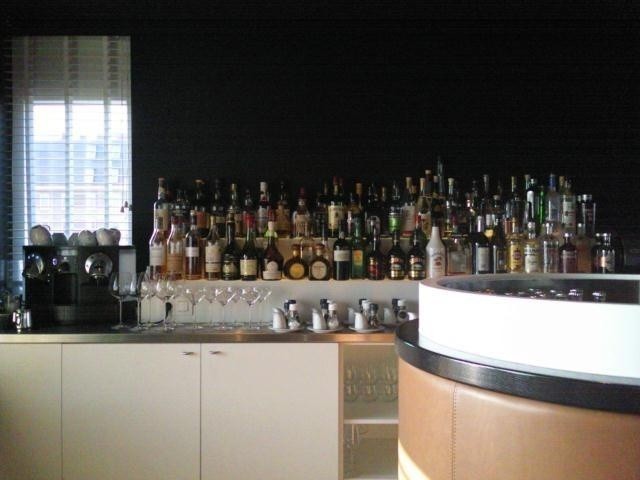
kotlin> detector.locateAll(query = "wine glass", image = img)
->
[107,271,271,334]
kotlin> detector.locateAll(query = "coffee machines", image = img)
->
[24,247,120,332]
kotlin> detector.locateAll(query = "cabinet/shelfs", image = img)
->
[138,230,606,326]
[0,343,62,479]
[61,344,339,480]
[341,334,400,480]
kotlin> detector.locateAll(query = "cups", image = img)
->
[12,309,32,333]
[1,295,23,315]
[26,224,122,245]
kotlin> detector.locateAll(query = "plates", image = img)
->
[267,319,416,334]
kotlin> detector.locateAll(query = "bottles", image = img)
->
[150,154,616,281]
